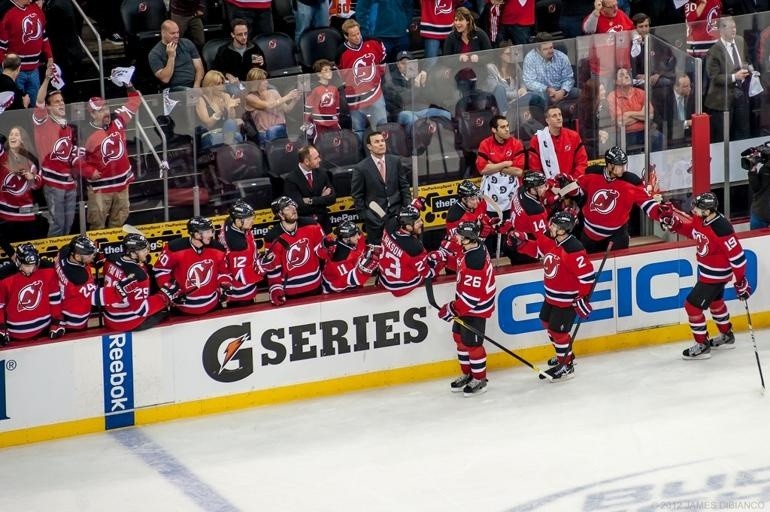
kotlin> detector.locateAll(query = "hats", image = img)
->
[87,97,109,113]
[396,51,412,60]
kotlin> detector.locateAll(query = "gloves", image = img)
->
[160,278,186,305]
[116,273,138,298]
[48,320,66,339]
[357,244,383,275]
[427,235,458,268]
[438,301,457,322]
[269,284,286,306]
[572,296,592,319]
[734,276,752,300]
[0,325,9,346]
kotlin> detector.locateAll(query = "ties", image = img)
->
[731,44,742,88]
[678,97,685,121]
[377,160,385,181]
[307,173,314,187]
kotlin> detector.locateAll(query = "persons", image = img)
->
[504,210,598,379]
[665,191,752,358]
[438,221,498,394]
[0,1,770,345]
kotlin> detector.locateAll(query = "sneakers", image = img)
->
[451,373,473,387]
[464,377,488,392]
[548,357,558,365]
[682,337,711,356]
[553,360,574,378]
[710,323,735,347]
